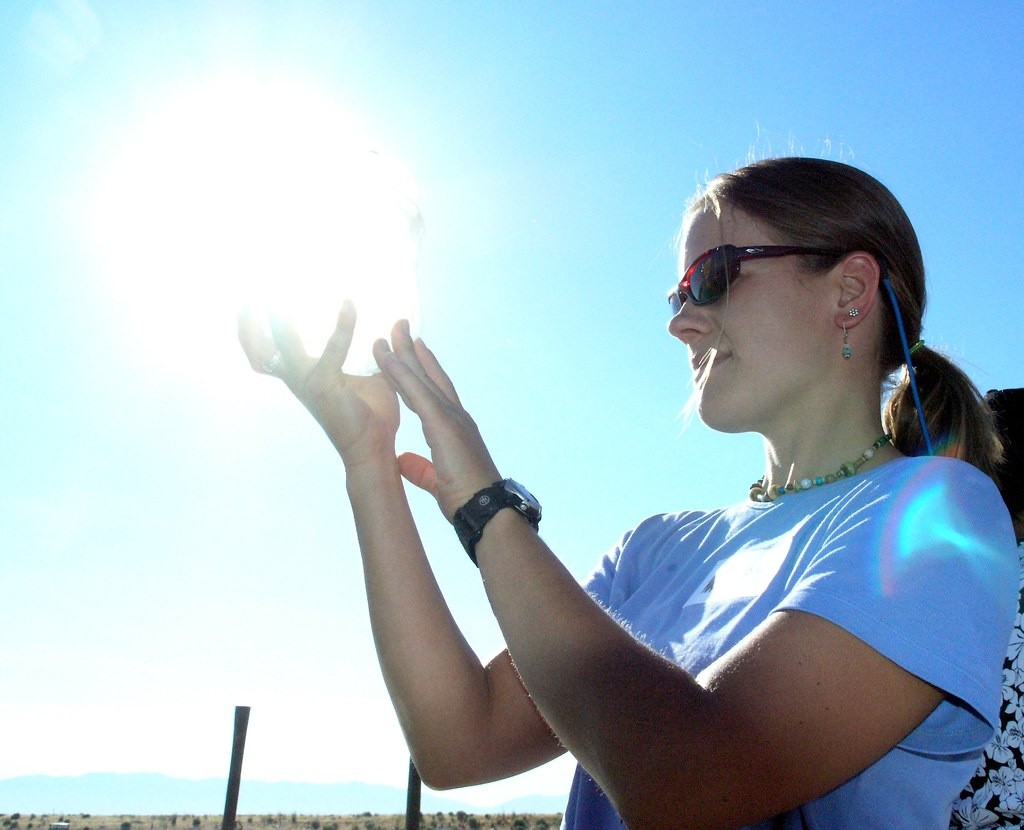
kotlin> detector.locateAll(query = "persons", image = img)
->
[239,157,1019,830]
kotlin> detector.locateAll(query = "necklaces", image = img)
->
[750,433,893,502]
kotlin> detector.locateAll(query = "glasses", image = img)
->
[669,241,846,315]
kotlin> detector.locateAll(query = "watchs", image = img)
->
[452,476,542,567]
